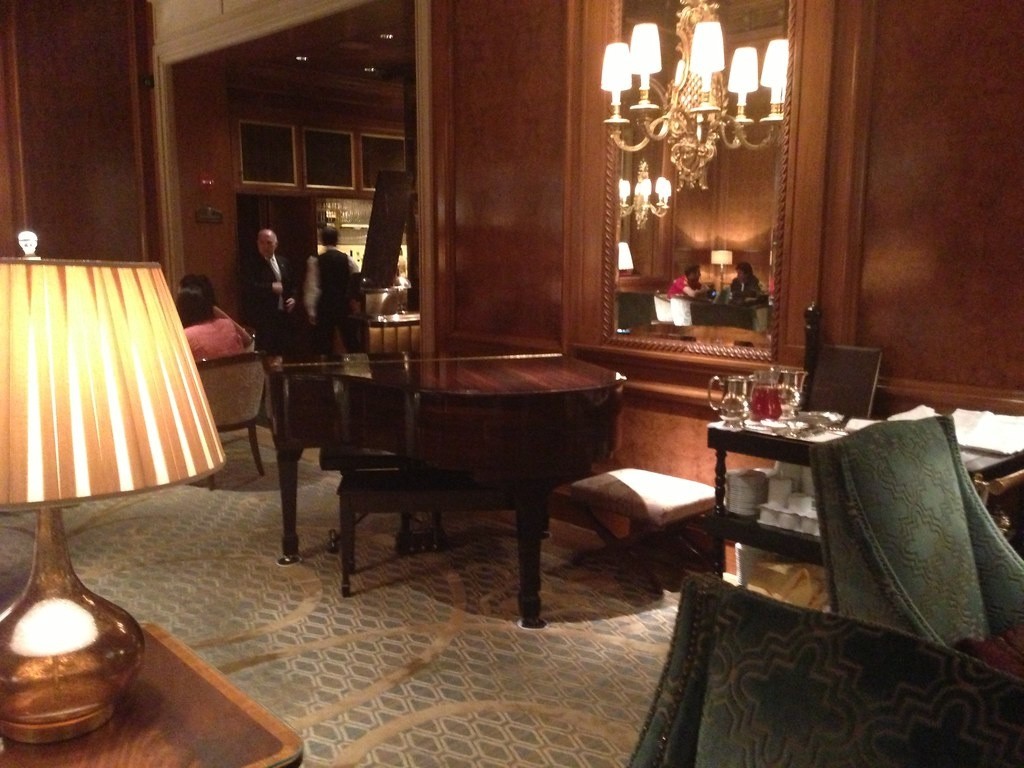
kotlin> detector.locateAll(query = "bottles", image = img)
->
[349,250,361,272]
[316,226,406,245]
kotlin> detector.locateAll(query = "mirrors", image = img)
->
[598,0,797,363]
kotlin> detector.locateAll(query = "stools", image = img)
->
[571,469,725,598]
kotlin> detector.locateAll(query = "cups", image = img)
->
[760,461,815,533]
[707,369,808,428]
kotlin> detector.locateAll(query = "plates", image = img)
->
[735,542,776,587]
[725,471,767,515]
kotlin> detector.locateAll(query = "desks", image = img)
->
[0,620,305,768]
[683,294,769,331]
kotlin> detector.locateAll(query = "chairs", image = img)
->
[623,569,1024,768]
[194,322,265,492]
[809,415,1024,685]
[361,287,398,315]
[654,282,774,332]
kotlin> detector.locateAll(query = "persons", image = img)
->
[236,228,296,354]
[176,289,244,362]
[177,274,251,349]
[303,227,362,356]
[730,261,760,297]
[667,263,709,300]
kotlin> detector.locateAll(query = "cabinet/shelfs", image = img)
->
[708,409,1024,579]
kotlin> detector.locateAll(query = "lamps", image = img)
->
[0,230,227,742]
[618,241,634,271]
[710,250,733,294]
[600,0,799,192]
[617,158,672,230]
